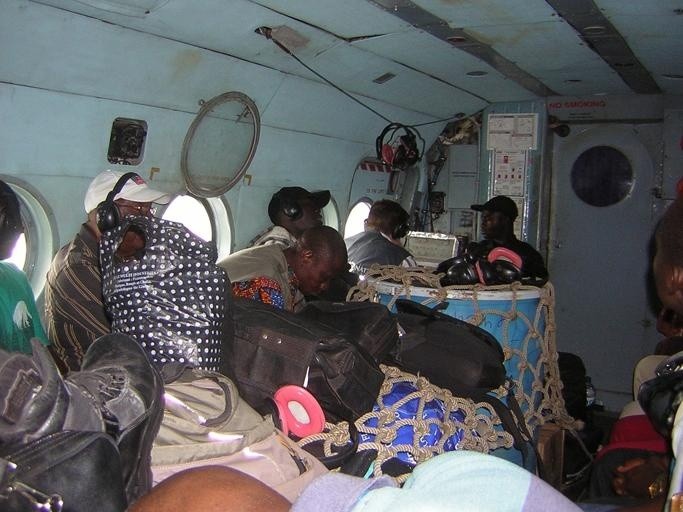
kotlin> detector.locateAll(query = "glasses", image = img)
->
[118,204,157,216]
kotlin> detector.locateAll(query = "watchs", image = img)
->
[647,472,669,499]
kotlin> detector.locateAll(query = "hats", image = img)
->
[470,196,518,217]
[268,187,331,225]
[84,169,171,214]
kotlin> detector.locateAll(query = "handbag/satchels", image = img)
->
[152,360,330,504]
[1,428,127,508]
[232,296,507,421]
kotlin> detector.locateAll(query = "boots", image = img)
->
[1,334,164,507]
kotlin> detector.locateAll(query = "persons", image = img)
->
[344,198,420,278]
[124,449,587,512]
[44,169,173,381]
[215,226,348,315]
[0,179,69,375]
[469,194,551,280]
[571,191,682,511]
[593,333,683,464]
[245,185,333,247]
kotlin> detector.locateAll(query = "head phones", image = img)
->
[254,383,360,465]
[433,255,476,287]
[277,186,304,220]
[96,171,144,234]
[377,122,424,169]
[364,199,411,240]
[477,246,550,287]
[0,179,25,245]
